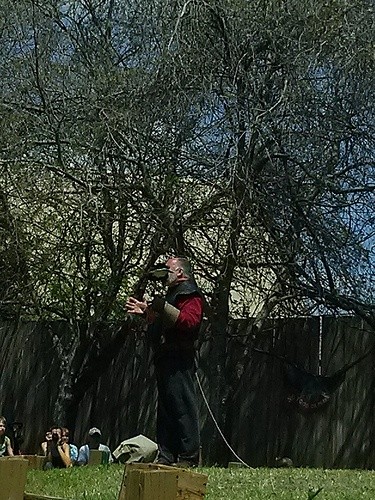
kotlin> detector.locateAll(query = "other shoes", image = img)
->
[155,458,199,468]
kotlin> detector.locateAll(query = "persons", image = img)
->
[0,414,114,469]
[122,255,203,469]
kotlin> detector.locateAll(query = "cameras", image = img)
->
[52,433,59,442]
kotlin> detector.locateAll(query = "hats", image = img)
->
[89,427,102,435]
